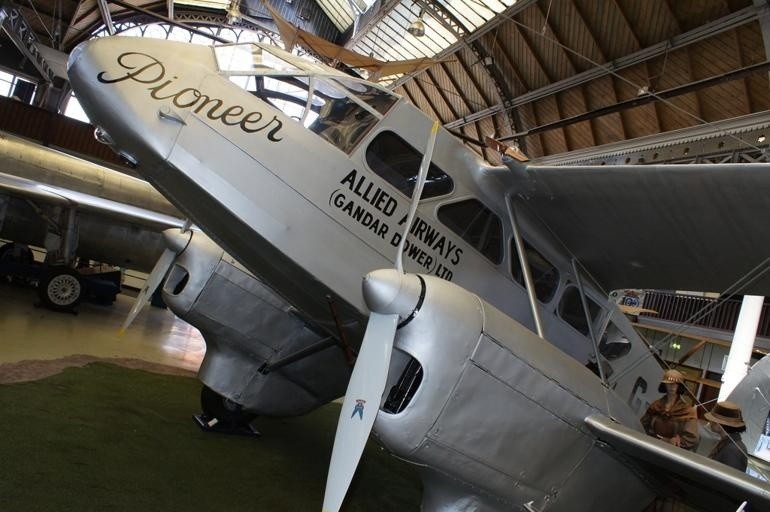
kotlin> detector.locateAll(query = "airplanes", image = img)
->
[66,36,770,512]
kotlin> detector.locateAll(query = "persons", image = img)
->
[639,370,699,450]
[703,402,748,472]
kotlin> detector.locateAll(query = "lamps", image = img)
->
[408,8,426,37]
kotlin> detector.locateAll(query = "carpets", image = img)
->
[1,351,423,511]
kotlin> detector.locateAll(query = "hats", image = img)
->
[659,368,686,385]
[703,402,746,427]
